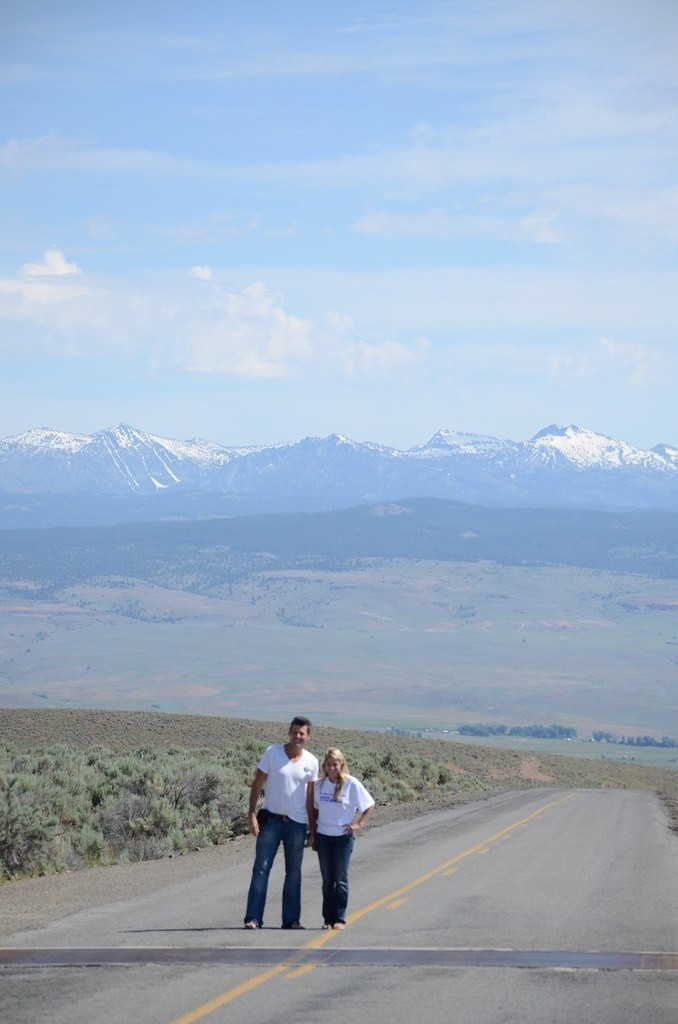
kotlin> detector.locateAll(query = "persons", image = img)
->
[309,746,375,931]
[243,717,318,930]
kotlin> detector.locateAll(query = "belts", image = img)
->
[268,813,291,822]
